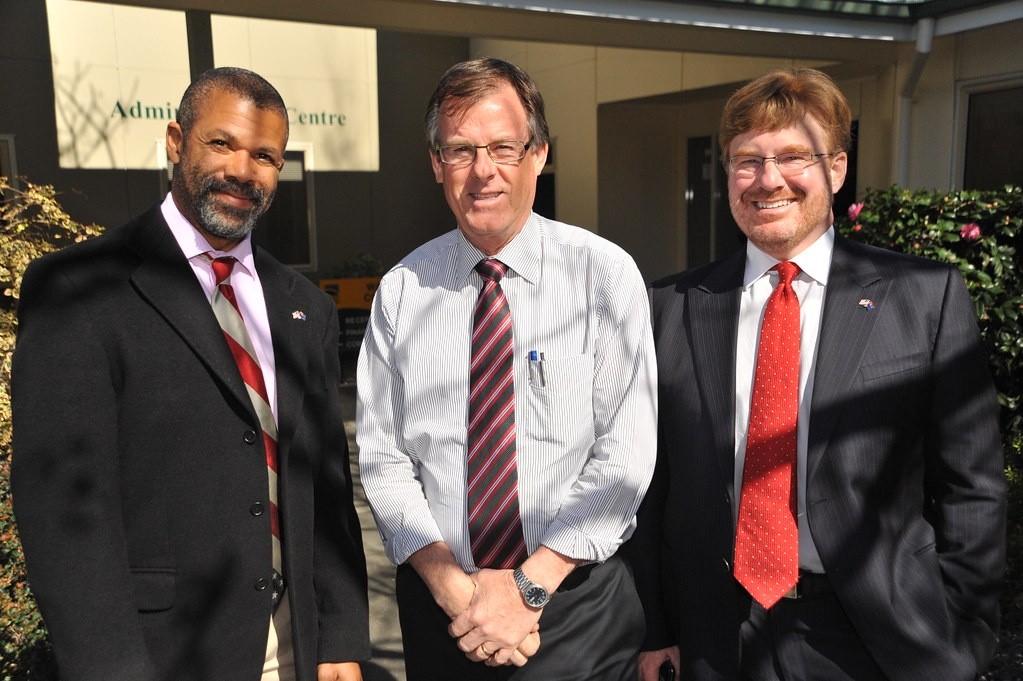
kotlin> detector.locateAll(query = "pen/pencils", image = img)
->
[540,353,546,385]
[528,350,537,381]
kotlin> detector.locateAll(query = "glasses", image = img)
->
[438,135,535,165]
[726,152,834,175]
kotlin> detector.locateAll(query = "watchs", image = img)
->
[514,567,552,609]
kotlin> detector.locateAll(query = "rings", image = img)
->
[481,644,492,656]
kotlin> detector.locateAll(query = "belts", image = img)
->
[781,573,825,599]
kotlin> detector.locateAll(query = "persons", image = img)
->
[10,67,370,681]
[636,67,1008,681]
[354,56,661,681]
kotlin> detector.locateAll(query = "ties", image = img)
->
[467,258,529,570]
[732,261,801,608]
[202,251,288,619]
